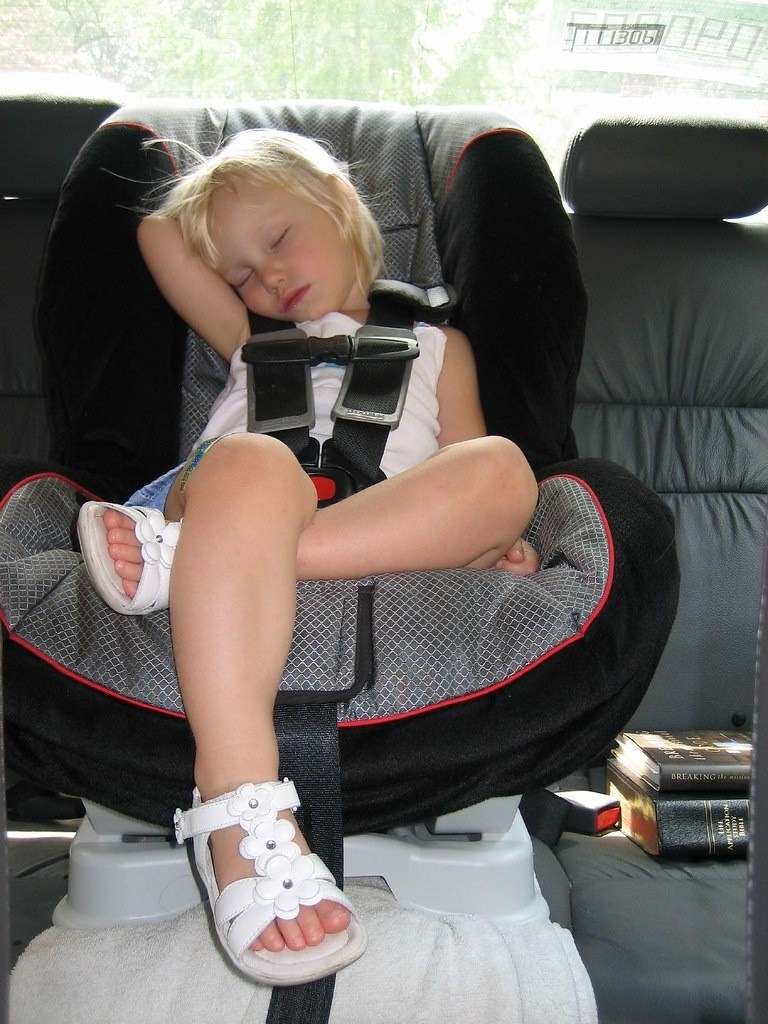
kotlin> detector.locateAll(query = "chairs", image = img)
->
[0,96,768,1024]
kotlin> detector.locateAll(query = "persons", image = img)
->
[76,129,540,987]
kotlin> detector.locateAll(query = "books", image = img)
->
[606,729,753,862]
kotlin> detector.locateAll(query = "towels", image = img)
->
[8,877,600,1024]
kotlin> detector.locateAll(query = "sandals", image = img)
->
[174,776,368,986]
[76,500,183,616]
